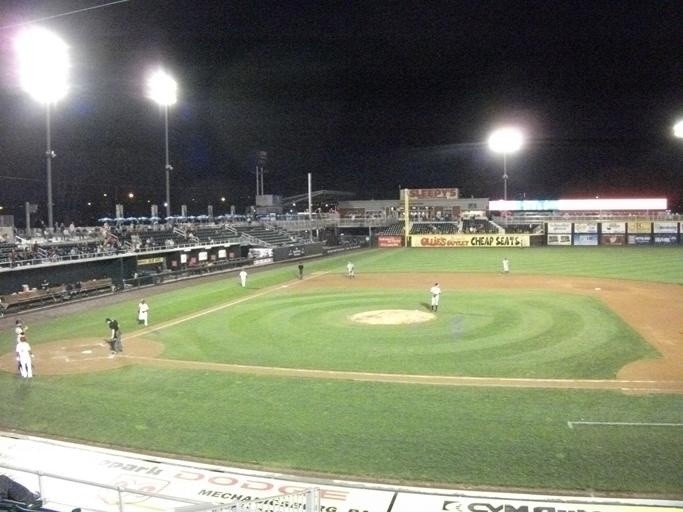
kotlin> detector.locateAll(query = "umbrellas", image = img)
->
[93,214,247,226]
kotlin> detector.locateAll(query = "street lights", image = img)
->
[148,66,178,216]
[491,125,516,202]
[17,23,71,226]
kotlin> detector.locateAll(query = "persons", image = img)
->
[73,281,81,291]
[298,261,304,279]
[13,336,34,378]
[346,260,355,279]
[14,319,27,338]
[136,298,148,326]
[104,318,122,354]
[14,331,24,343]
[502,257,509,272]
[65,283,75,294]
[58,282,65,303]
[0,219,188,265]
[239,269,247,287]
[39,279,49,291]
[428,281,441,312]
